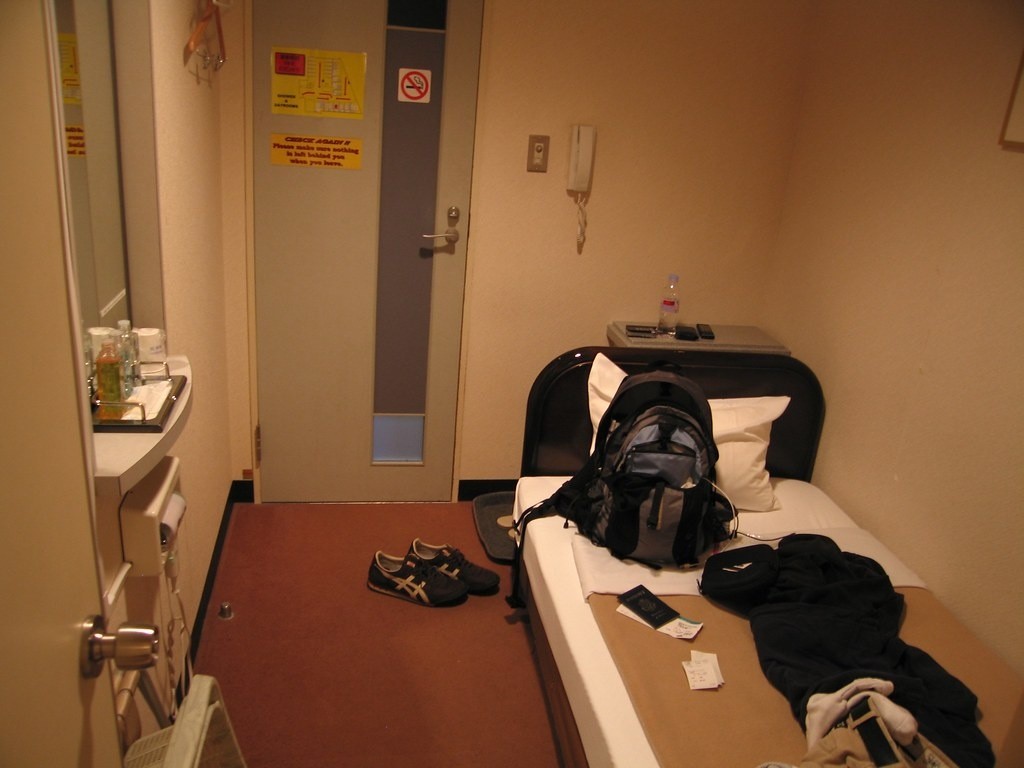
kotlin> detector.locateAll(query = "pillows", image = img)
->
[586,352,793,513]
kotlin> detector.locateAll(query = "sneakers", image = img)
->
[366,550,470,608]
[407,538,501,595]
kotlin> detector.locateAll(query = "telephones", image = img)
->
[567,124,594,192]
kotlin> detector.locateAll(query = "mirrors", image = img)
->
[52,0,133,408]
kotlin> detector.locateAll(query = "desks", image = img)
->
[609,321,793,356]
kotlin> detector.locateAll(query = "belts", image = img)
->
[850,697,900,768]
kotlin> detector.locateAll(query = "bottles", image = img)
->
[117,319,141,388]
[90,326,112,368]
[657,273,681,338]
[109,329,132,399]
[95,338,124,421]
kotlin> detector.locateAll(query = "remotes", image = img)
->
[697,324,715,339]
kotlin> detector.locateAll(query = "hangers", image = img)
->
[182,0,228,71]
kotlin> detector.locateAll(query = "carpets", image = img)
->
[471,490,518,560]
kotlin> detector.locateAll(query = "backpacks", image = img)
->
[513,372,740,568]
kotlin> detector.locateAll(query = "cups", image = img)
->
[136,327,167,374]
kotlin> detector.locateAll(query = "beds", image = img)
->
[507,345,1024,768]
